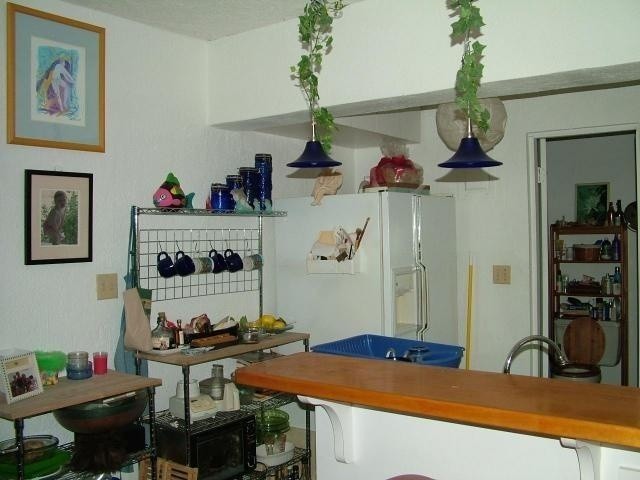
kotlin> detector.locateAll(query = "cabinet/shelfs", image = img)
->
[138,324,311,480]
[549,226,638,390]
[0,368,162,480]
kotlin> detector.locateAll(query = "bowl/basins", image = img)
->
[0,434,59,463]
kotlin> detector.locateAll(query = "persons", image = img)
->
[11,371,33,397]
[43,191,68,245]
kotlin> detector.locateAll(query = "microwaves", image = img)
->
[145,414,257,480]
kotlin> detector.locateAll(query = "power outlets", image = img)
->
[493,265,512,284]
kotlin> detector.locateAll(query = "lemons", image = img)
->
[260,315,276,327]
[272,321,286,329]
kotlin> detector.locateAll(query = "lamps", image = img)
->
[286,23,344,169]
[436,28,503,170]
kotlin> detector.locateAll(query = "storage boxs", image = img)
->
[312,333,466,368]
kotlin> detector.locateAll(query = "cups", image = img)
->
[92,351,108,375]
[224,249,243,273]
[192,249,214,274]
[209,249,226,274]
[242,248,263,271]
[156,251,176,278]
[175,250,196,278]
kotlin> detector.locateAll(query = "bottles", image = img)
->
[604,273,612,295]
[600,237,612,261]
[613,267,622,296]
[612,233,621,261]
[615,200,624,226]
[607,202,615,227]
[562,273,568,295]
[556,270,562,293]
[608,299,613,320]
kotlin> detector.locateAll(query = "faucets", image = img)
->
[502,336,569,377]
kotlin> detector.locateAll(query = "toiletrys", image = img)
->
[615,267,621,283]
[611,299,617,320]
[556,271,562,291]
[562,216,565,229]
[601,277,606,294]
[609,299,612,319]
[562,276,568,294]
[605,273,611,296]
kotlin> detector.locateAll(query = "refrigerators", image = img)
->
[275,193,459,356]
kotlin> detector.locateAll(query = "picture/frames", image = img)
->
[575,183,610,225]
[6,3,108,155]
[23,168,95,264]
